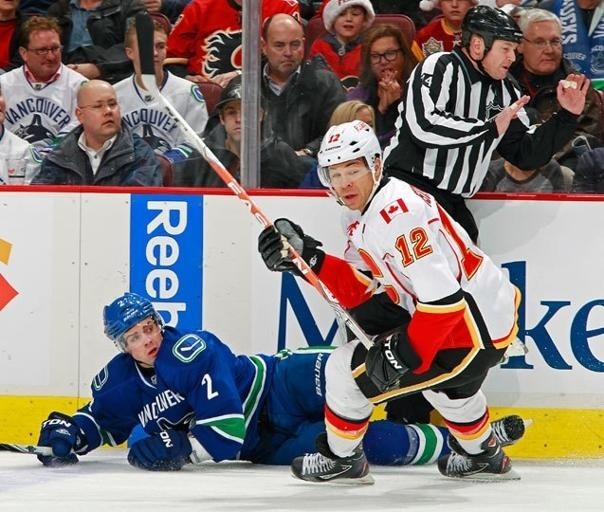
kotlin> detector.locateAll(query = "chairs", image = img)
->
[154,153,174,187]
[371,14,416,52]
[191,82,224,118]
[149,13,171,39]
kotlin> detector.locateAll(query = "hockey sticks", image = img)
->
[135,13,375,350]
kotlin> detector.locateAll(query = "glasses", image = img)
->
[523,35,563,51]
[26,44,63,56]
[368,48,402,66]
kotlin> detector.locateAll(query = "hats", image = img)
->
[215,83,265,109]
[320,0,375,35]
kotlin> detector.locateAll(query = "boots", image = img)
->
[490,415,524,447]
[437,431,512,477]
[290,432,369,483]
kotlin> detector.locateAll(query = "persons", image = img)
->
[1,0,603,195]
[37,293,525,471]
[260,120,521,484]
[381,1,592,424]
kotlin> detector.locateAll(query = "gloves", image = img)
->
[258,218,325,277]
[37,411,80,467]
[364,331,422,393]
[126,429,192,471]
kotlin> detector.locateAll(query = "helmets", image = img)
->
[316,119,384,206]
[460,5,524,51]
[102,292,166,355]
